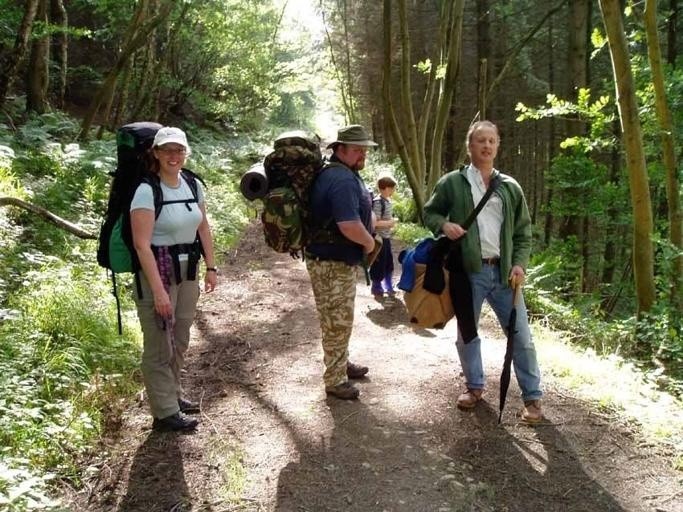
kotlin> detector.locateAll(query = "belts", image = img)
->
[481,259,499,265]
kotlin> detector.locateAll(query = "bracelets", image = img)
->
[207,267,217,272]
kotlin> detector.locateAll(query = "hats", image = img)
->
[327,124,380,149]
[151,124,190,149]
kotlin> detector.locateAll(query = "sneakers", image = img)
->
[347,361,369,379]
[521,400,544,423]
[456,386,483,409]
[179,398,201,414]
[153,414,198,431]
[373,290,399,303]
[325,382,359,400]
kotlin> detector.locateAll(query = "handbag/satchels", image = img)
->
[403,257,456,330]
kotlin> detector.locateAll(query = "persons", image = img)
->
[129,126,217,432]
[369,178,399,297]
[421,120,543,424]
[302,125,377,399]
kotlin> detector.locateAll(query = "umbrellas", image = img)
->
[498,273,521,425]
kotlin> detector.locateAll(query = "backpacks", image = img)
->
[255,129,357,253]
[96,120,206,273]
